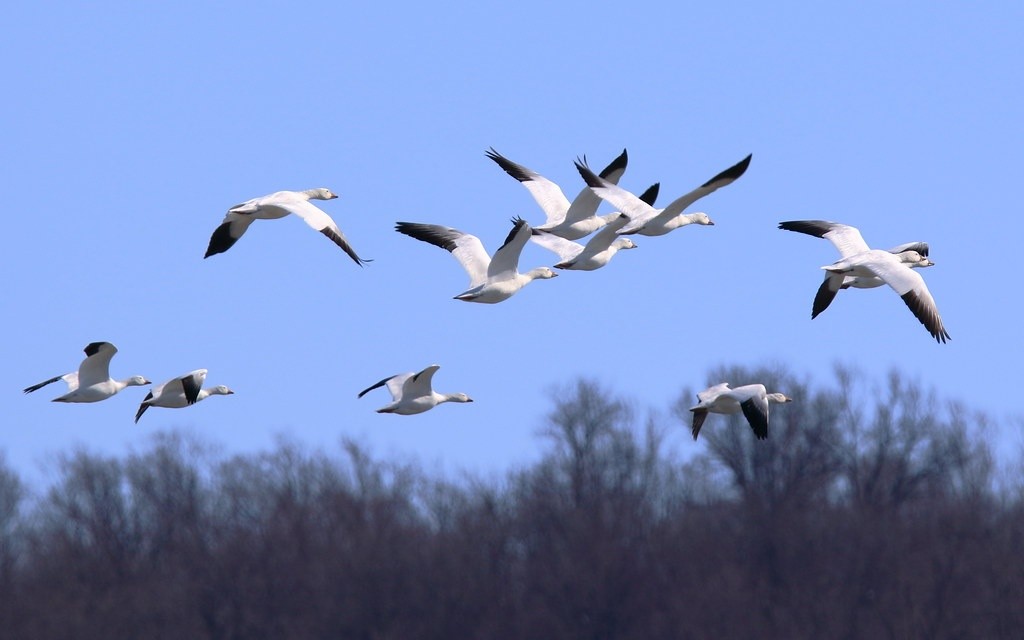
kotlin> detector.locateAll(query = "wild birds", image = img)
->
[689,381,793,442]
[22,341,152,404]
[484,145,623,241]
[204,188,375,270]
[573,153,752,237]
[393,214,559,305]
[777,219,952,345]
[511,181,661,271]
[134,368,234,424]
[357,364,473,415]
